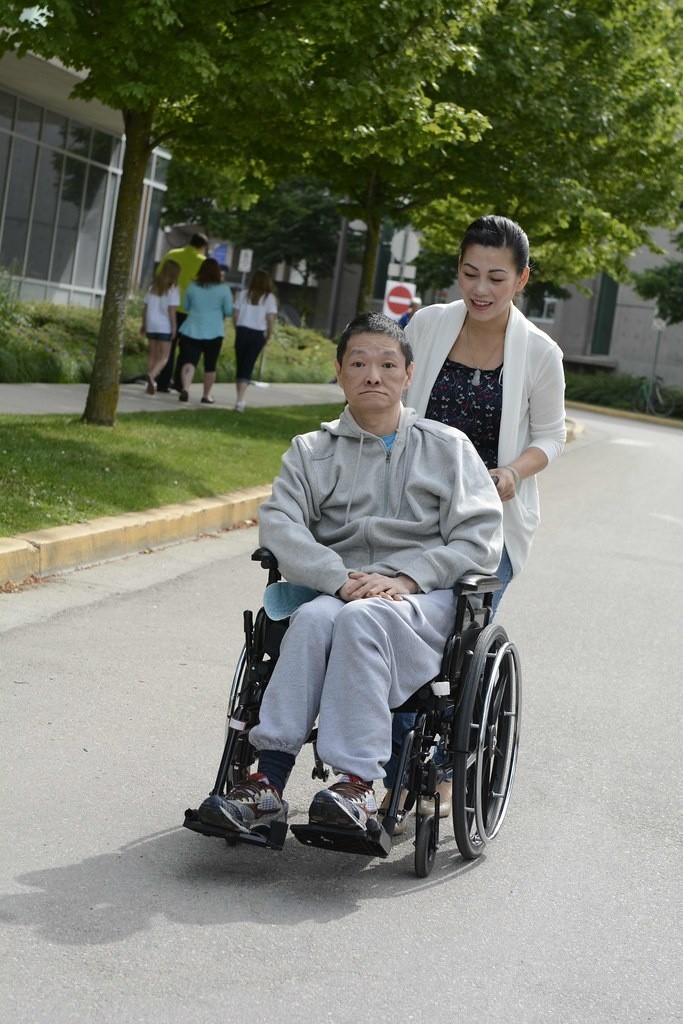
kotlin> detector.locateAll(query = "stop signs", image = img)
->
[382,280,416,322]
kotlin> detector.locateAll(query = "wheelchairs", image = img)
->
[212,545,526,879]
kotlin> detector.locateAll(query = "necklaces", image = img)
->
[464,318,504,387]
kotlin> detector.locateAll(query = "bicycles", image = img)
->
[633,374,677,418]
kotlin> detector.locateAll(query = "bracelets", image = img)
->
[503,462,521,490]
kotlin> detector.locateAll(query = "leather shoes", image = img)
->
[376,788,411,834]
[418,777,454,817]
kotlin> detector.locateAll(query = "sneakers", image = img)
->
[201,396,216,404]
[179,390,189,402]
[308,773,378,828]
[201,773,290,837]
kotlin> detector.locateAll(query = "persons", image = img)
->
[139,260,180,394]
[397,297,421,328]
[176,258,233,404]
[377,216,566,834]
[199,312,505,835]
[151,233,210,394]
[231,267,279,410]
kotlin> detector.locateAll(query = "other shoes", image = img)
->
[169,382,183,391]
[234,400,246,412]
[144,372,157,394]
[157,387,170,393]
